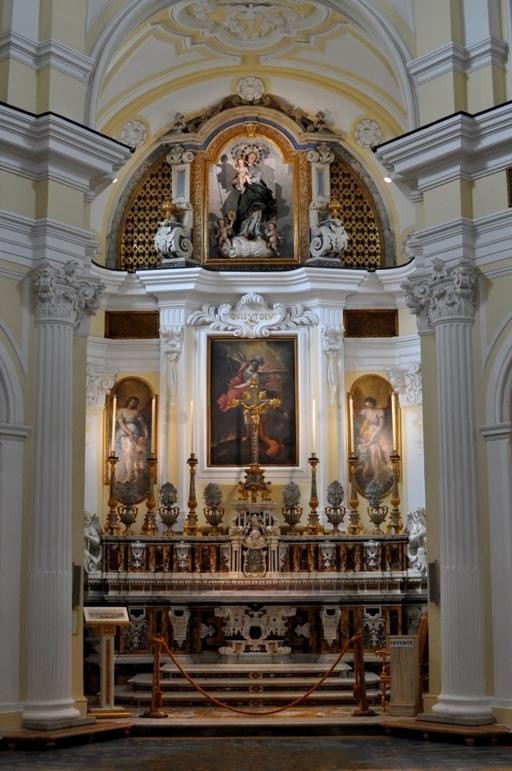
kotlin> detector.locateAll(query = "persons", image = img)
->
[130,435,148,481]
[234,150,277,238]
[232,157,251,186]
[265,222,283,256]
[108,395,148,481]
[211,216,235,248]
[217,354,264,441]
[353,397,391,478]
[356,436,371,478]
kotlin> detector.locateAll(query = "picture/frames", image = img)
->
[191,122,312,266]
[206,334,300,468]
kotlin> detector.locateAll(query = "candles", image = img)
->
[311,399,316,454]
[391,391,397,451]
[191,402,195,453]
[150,395,156,454]
[111,394,117,452]
[349,395,355,453]
